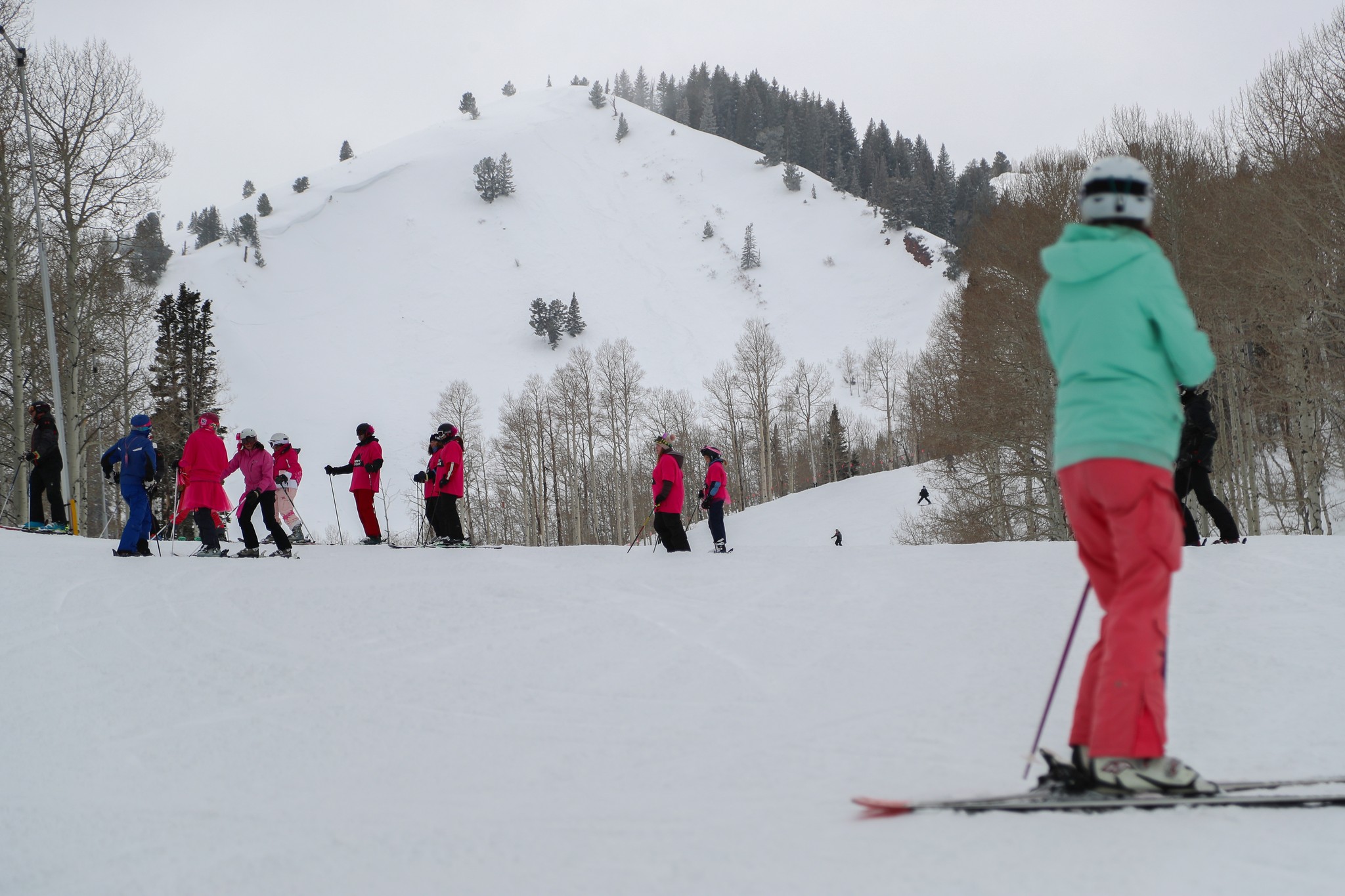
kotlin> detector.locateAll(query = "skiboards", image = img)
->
[0,519,74,536]
[237,536,339,545]
[387,542,503,549]
[852,748,1345,817]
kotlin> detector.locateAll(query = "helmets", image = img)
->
[30,401,50,421]
[1080,155,1153,224]
[356,423,374,438]
[240,428,257,440]
[1177,379,1198,391]
[702,447,721,461]
[268,433,289,445]
[198,412,220,429]
[437,423,458,438]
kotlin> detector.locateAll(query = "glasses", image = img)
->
[700,445,721,454]
[356,429,366,435]
[269,440,283,449]
[437,430,452,438]
[654,436,672,448]
[29,405,41,414]
[242,435,257,446]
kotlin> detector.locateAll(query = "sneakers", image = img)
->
[359,537,382,545]
[197,545,221,557]
[269,548,292,558]
[39,522,70,534]
[1183,539,1201,547]
[423,536,470,547]
[22,521,45,530]
[1211,537,1241,544]
[236,547,259,557]
[1071,743,1221,797]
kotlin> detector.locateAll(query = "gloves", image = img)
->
[365,463,376,472]
[144,478,156,493]
[324,464,333,475]
[655,494,665,503]
[174,460,180,468]
[25,452,36,462]
[104,472,119,485]
[1189,443,1209,466]
[275,474,288,485]
[245,489,261,505]
[413,474,425,484]
[439,474,449,488]
[418,471,426,480]
[701,499,711,510]
[699,490,704,498]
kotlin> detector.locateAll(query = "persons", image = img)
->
[432,423,468,545]
[325,423,384,545]
[652,433,691,552]
[832,529,843,546]
[1172,384,1242,545]
[263,433,306,542]
[100,413,233,558]
[21,399,70,533]
[219,427,293,558]
[698,444,731,553]
[413,433,446,545]
[917,486,932,505]
[1036,155,1220,797]
[942,452,957,473]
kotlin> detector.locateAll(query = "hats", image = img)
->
[430,434,441,442]
[129,415,152,435]
[655,434,675,450]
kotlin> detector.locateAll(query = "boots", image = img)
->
[217,528,228,541]
[261,533,275,544]
[136,537,152,557]
[115,548,143,557]
[165,524,179,539]
[287,526,305,543]
[708,538,726,553]
